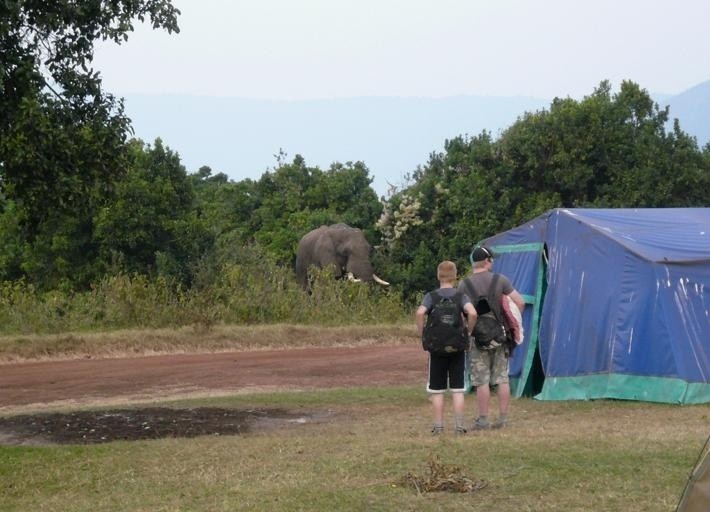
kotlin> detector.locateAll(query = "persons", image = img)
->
[457,246,526,432]
[414,260,478,439]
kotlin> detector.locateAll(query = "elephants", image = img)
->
[294,221,391,297]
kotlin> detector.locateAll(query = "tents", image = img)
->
[461,206,708,406]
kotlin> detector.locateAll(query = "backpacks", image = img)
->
[463,273,514,350]
[421,290,471,356]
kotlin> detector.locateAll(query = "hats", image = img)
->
[470,246,501,262]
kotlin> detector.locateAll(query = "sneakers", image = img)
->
[430,417,510,436]
